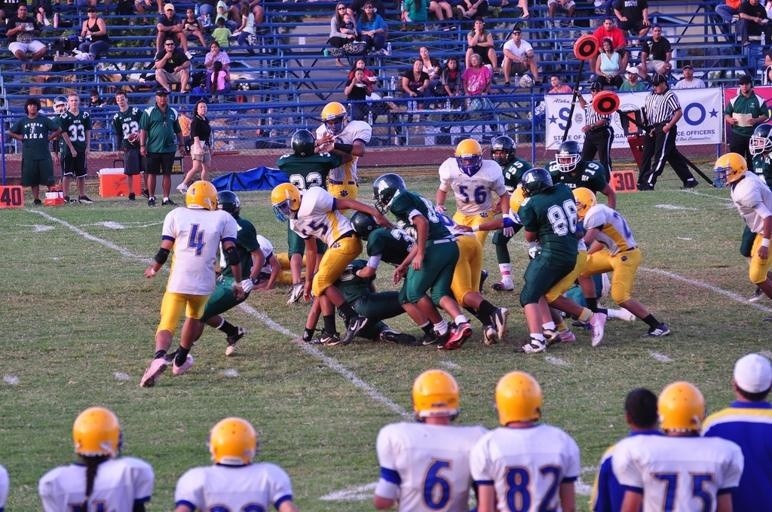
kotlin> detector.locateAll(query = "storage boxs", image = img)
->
[48,184,64,191]
[45,192,64,199]
[97,168,142,197]
[44,199,64,207]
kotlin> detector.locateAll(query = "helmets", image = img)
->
[321,102,347,132]
[713,123,772,186]
[453,134,597,220]
[655,379,707,432]
[350,206,382,243]
[186,180,241,210]
[209,415,258,466]
[53,96,69,115]
[495,370,543,424]
[72,404,120,460]
[412,368,459,421]
[372,173,408,215]
[271,183,301,221]
[291,129,314,154]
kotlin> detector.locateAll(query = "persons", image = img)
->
[170,415,298,511]
[140,101,672,386]
[696,348,771,511]
[713,153,766,301]
[587,386,661,511]
[374,367,498,511]
[37,405,156,511]
[468,371,581,511]
[608,378,745,511]
[2,0,767,208]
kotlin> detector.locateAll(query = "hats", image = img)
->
[733,352,772,394]
[513,27,522,35]
[628,65,694,85]
[164,3,176,11]
[156,89,171,96]
[736,74,754,85]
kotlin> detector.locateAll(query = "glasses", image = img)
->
[336,5,373,10]
[165,41,176,47]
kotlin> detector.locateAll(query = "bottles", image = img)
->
[53,13,58,28]
[46,189,64,199]
[761,31,765,46]
[391,76,395,89]
[407,99,418,110]
[56,51,60,56]
[324,48,330,56]
[367,111,374,125]
[205,14,212,26]
[194,3,199,16]
[445,98,452,109]
[514,73,519,86]
[48,43,52,57]
[387,42,392,54]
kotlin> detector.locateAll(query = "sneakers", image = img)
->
[31,183,188,206]
[491,280,515,291]
[618,307,636,324]
[646,323,672,337]
[223,324,245,354]
[139,355,194,388]
[286,283,304,306]
[586,313,606,347]
[302,307,513,354]
[522,307,577,351]
[636,178,699,191]
